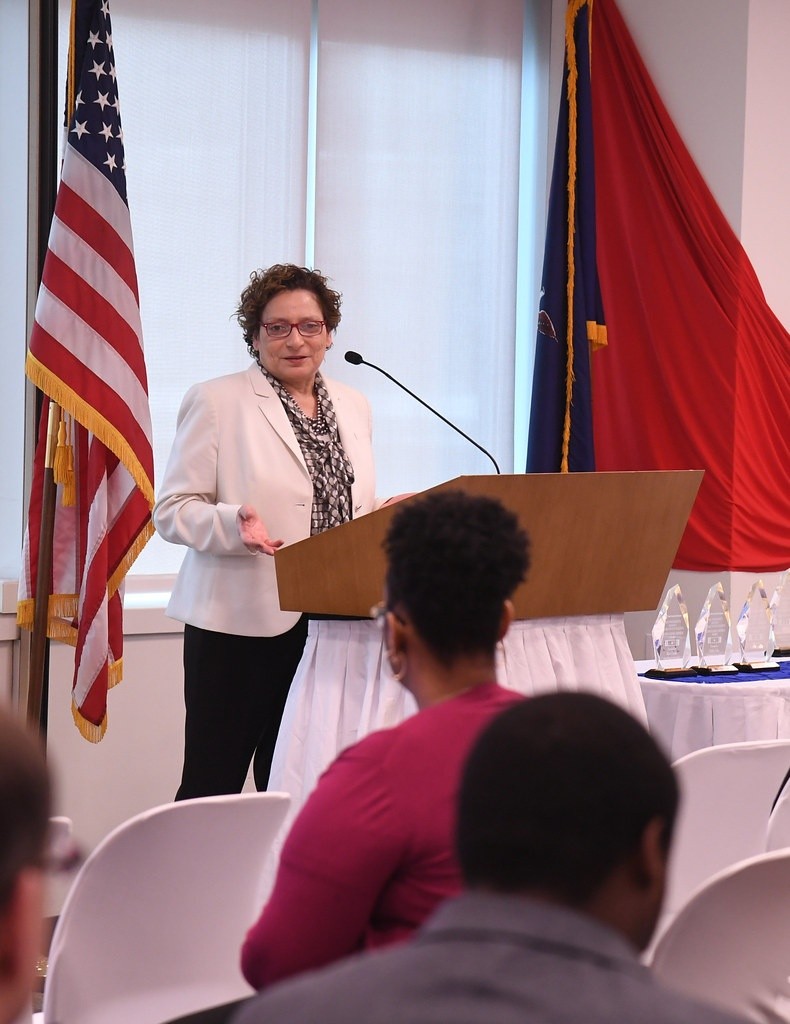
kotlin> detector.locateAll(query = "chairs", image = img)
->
[645,851,790,1024]
[32,789,289,1024]
[642,739,790,963]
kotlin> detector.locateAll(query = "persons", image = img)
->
[0,705,53,1024]
[151,262,419,802]
[239,488,533,994]
[231,689,759,1024]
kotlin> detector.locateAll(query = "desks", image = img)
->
[633,651,790,764]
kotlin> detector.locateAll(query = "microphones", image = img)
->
[344,351,500,474]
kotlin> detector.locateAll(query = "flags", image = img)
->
[525,0,790,573]
[15,0,157,744]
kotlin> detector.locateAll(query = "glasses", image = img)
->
[368,599,407,628]
[251,319,326,339]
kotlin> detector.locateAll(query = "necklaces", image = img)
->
[283,386,328,434]
